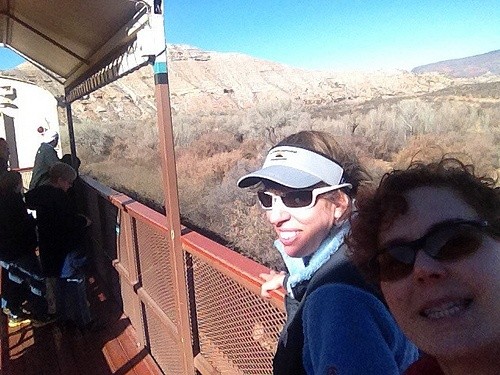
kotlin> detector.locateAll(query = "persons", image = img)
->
[235,131,420,375]
[25,130,106,334]
[343,146,500,375]
[0,138,58,327]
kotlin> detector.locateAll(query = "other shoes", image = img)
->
[33,313,58,327]
[8,314,33,327]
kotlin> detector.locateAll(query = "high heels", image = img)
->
[58,317,79,335]
[79,319,106,336]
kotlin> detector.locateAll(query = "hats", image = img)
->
[42,129,58,143]
[237,146,359,198]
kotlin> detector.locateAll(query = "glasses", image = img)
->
[256,183,351,210]
[369,219,492,284]
[67,179,73,187]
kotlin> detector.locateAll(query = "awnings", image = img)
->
[0,0,182,106]
